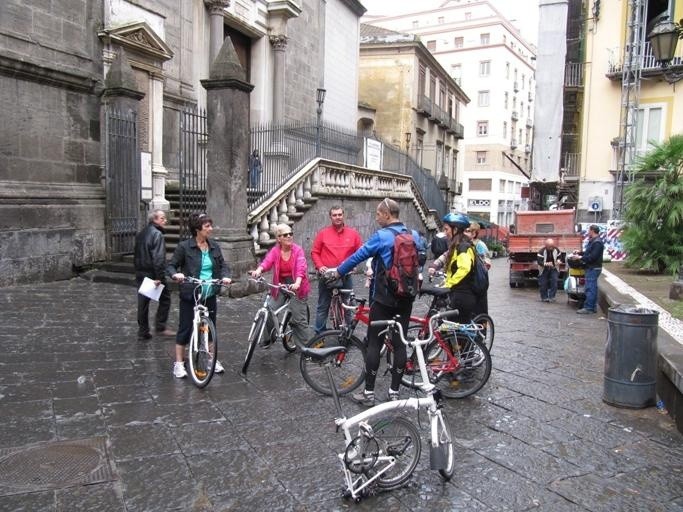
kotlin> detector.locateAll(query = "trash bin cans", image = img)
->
[603,307,660,409]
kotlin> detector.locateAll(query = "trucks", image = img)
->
[507,206,583,288]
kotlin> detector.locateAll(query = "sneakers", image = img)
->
[261,335,276,349]
[138,333,152,340]
[577,308,593,314]
[173,361,188,378]
[349,391,376,408]
[155,329,176,337]
[312,339,326,349]
[387,387,400,402]
[549,297,556,302]
[207,359,225,374]
[542,298,549,302]
[593,309,598,313]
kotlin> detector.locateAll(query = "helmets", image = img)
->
[439,212,470,228]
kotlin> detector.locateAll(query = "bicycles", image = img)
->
[166,272,234,389]
[240,270,309,370]
[301,272,493,400]
[301,310,462,507]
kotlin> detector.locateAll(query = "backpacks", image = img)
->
[375,224,420,303]
[457,241,489,298]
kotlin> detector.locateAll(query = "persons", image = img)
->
[133,208,176,341]
[311,205,362,339]
[166,212,233,380]
[537,238,562,303]
[571,224,603,315]
[335,198,421,407]
[248,150,262,190]
[250,223,311,358]
[418,211,491,382]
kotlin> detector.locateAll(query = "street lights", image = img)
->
[313,87,326,158]
